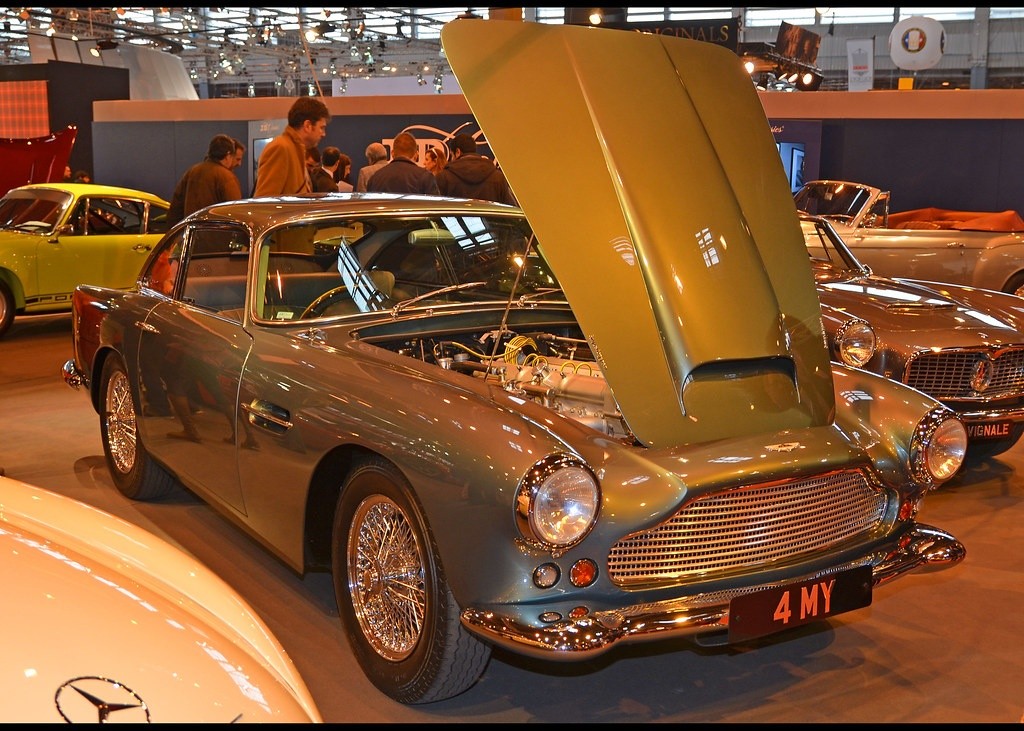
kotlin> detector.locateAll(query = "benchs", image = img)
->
[163,272,395,314]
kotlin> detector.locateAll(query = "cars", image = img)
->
[0,181,173,341]
[60,15,1024,709]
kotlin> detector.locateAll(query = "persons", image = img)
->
[366,132,440,196]
[166,135,244,235]
[424,147,448,177]
[74,170,90,183]
[253,96,330,253]
[434,133,517,207]
[357,142,388,193]
[305,146,355,193]
[63,165,71,182]
